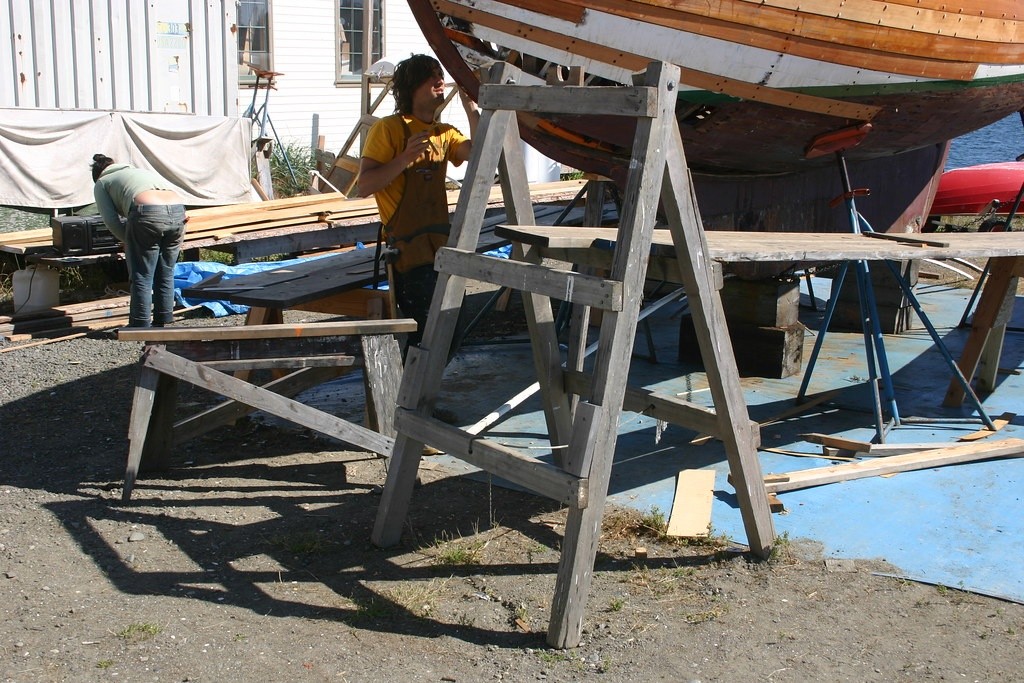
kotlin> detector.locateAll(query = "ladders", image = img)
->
[370,60,780,651]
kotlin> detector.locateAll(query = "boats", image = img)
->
[402,0,1024,180]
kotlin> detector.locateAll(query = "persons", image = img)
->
[92,154,185,338]
[358,55,473,368]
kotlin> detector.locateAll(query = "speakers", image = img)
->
[52,217,88,256]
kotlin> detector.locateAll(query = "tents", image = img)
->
[0,107,253,211]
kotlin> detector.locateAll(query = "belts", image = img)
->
[130,204,184,212]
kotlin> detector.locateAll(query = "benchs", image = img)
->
[117,318,419,503]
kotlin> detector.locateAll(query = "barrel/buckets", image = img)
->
[12,265,61,312]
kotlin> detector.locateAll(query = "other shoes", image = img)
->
[150,321,175,327]
[422,443,437,455]
[430,407,457,424]
[113,325,131,338]
